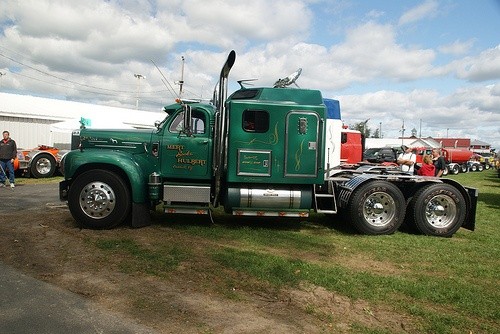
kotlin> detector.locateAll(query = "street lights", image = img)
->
[133,73,146,124]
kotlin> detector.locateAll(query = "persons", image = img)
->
[491,161,494,170]
[397,145,416,175]
[0,131,17,188]
[430,149,444,177]
[417,155,435,176]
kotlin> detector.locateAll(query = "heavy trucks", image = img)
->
[58,50,481,238]
[322,98,500,178]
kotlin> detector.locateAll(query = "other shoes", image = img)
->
[0,183,5,187]
[10,182,15,188]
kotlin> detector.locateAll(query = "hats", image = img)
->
[405,145,413,149]
